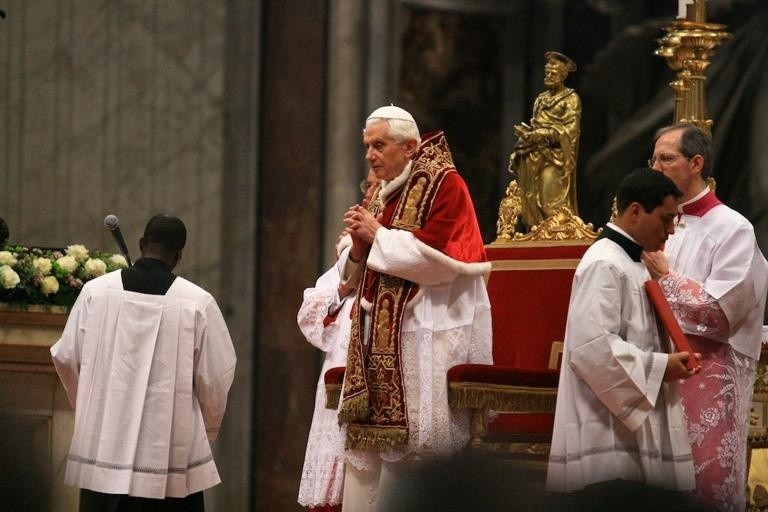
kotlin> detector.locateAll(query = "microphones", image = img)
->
[103,213,131,265]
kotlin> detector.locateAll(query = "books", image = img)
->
[642,277,698,372]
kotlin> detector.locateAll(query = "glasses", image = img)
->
[646,152,703,168]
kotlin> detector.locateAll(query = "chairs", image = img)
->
[324,340,564,512]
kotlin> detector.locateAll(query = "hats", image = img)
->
[366,103,416,123]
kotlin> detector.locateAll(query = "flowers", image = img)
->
[0,243,134,311]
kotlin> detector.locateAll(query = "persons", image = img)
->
[493,48,586,245]
[539,168,706,499]
[338,102,496,511]
[606,121,768,511]
[295,166,384,510]
[47,213,238,510]
